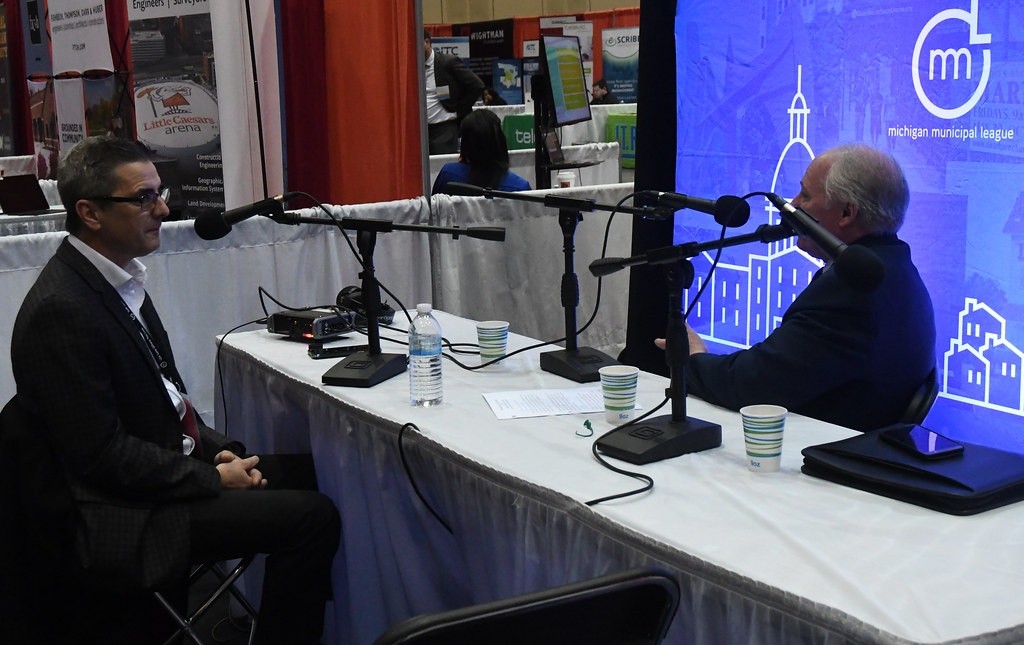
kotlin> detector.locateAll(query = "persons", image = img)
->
[423,29,486,155]
[655,142,937,433]
[482,87,508,106]
[590,79,620,105]
[10,135,343,645]
[431,109,532,196]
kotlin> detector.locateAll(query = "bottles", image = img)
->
[408,304,442,407]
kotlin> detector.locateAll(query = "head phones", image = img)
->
[335,286,395,325]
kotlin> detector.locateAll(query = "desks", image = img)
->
[213,308,1024,645]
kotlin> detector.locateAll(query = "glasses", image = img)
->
[78,186,170,211]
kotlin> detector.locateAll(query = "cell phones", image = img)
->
[880,423,965,461]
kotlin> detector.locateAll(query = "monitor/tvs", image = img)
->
[540,34,593,126]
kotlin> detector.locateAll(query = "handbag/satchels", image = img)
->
[801,426,1024,516]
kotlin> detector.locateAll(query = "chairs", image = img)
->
[0,394,272,645]
[892,359,939,428]
[377,569,681,645]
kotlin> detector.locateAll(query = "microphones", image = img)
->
[641,190,750,228]
[767,192,884,289]
[194,190,301,240]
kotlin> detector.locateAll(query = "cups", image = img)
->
[740,405,788,473]
[597,365,639,424]
[476,321,509,368]
[557,172,576,188]
